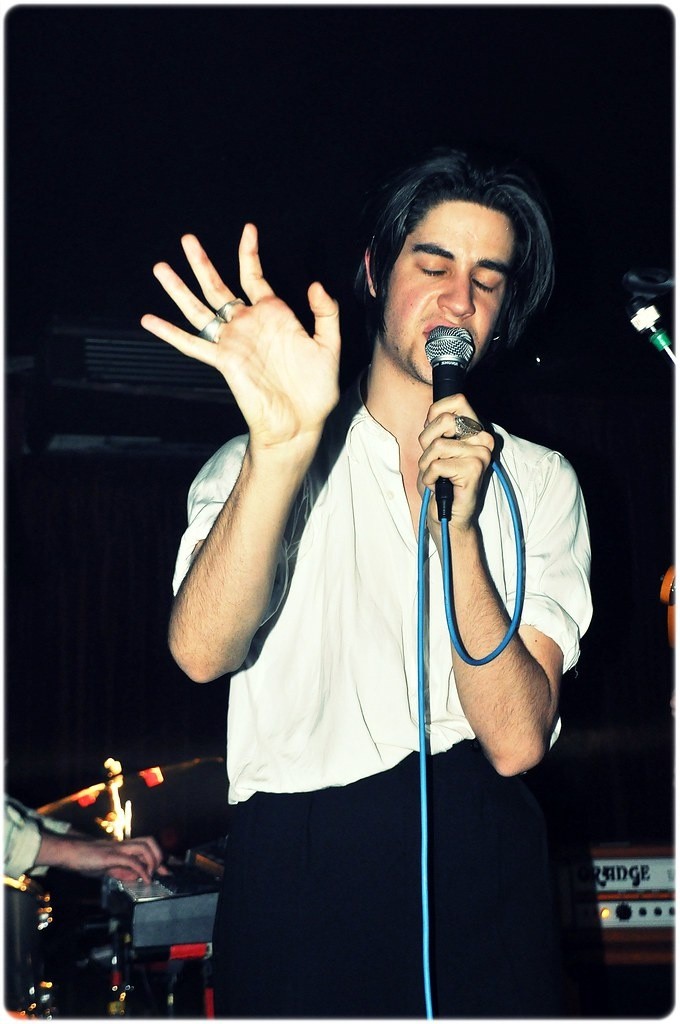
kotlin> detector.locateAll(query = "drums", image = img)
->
[5,870,58,1018]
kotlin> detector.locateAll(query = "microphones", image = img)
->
[425,325,476,521]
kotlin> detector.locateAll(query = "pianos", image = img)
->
[98,839,232,950]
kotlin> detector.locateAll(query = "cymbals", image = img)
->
[33,752,225,823]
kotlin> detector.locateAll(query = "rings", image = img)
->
[217,298,245,319]
[197,315,226,340]
[451,415,485,441]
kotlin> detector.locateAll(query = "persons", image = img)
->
[140,153,596,1019]
[3,793,170,887]
